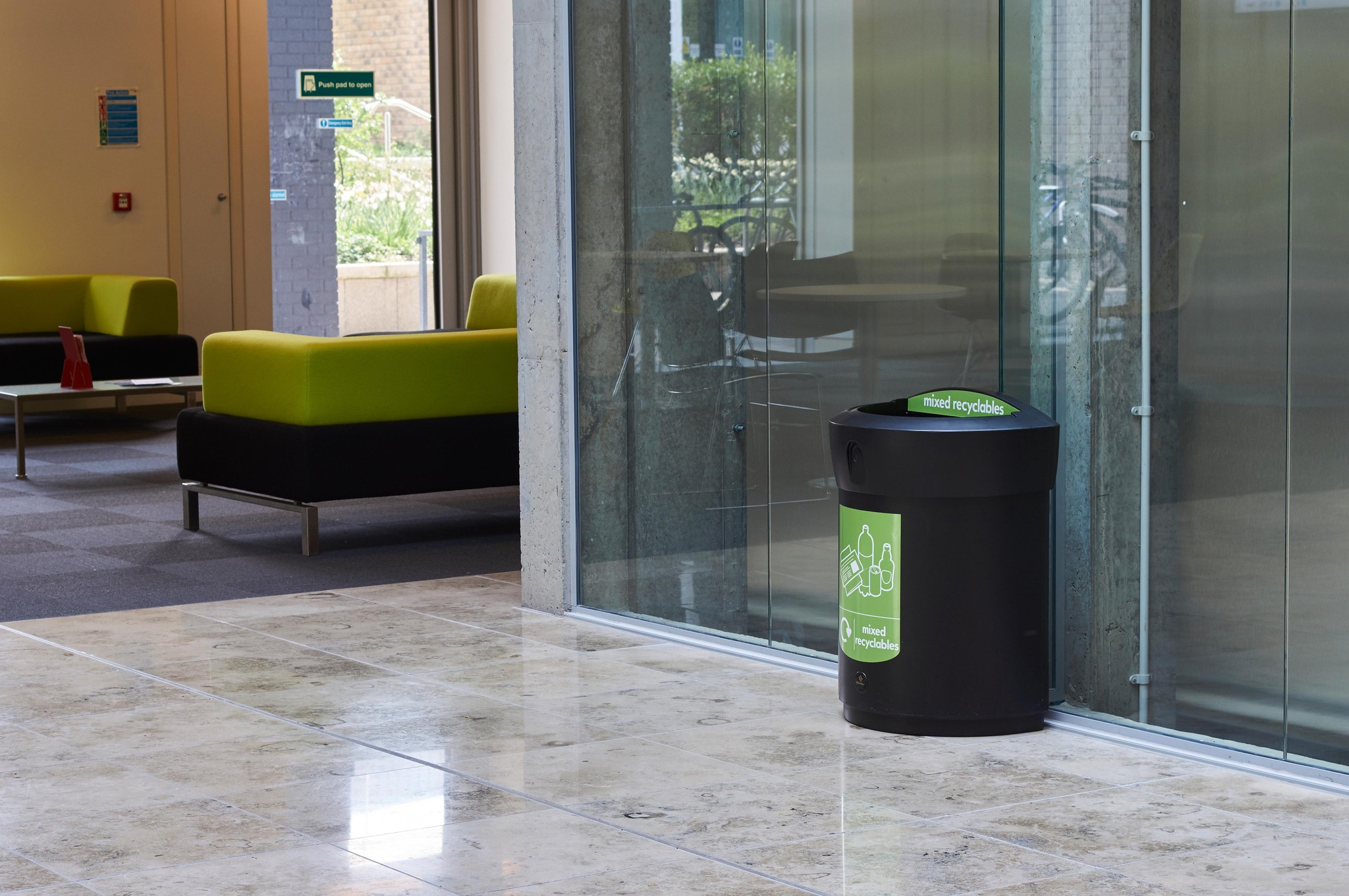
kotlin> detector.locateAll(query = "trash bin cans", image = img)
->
[828,387,1060,737]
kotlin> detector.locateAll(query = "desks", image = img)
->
[758,284,966,356]
[1,371,203,478]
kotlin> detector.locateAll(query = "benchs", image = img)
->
[176,274,520,555]
[717,244,856,357]
[1,276,199,415]
